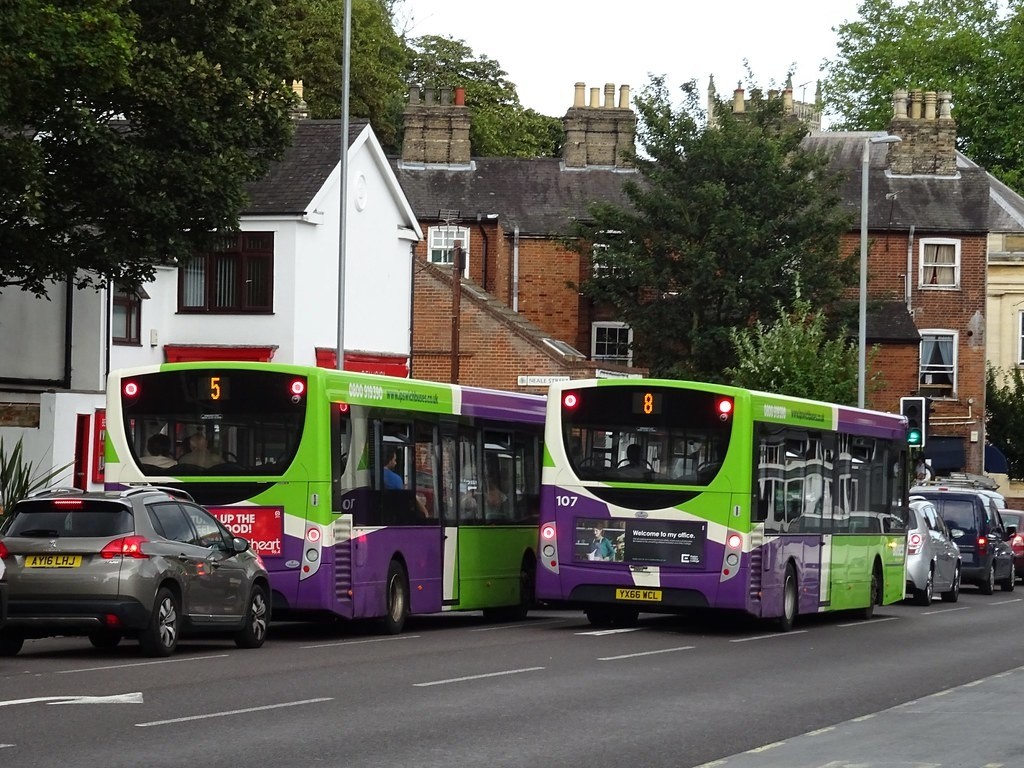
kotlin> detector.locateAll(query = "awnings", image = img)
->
[925,436,966,469]
[985,444,1007,473]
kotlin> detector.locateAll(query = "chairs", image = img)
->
[697,462,722,484]
[73,514,116,534]
[220,451,242,466]
[27,515,65,532]
[575,458,653,476]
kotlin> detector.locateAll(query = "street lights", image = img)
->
[856,135,904,410]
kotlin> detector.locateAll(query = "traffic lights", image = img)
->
[899,397,928,450]
[923,395,936,434]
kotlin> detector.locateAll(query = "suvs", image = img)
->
[0,483,272,660]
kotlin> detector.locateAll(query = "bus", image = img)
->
[534,375,925,635]
[100,361,546,637]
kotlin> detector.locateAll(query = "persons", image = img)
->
[381,447,404,489]
[915,456,926,479]
[138,433,178,470]
[177,433,227,468]
[589,521,616,561]
[618,443,652,480]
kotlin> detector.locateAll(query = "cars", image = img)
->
[902,480,1024,607]
